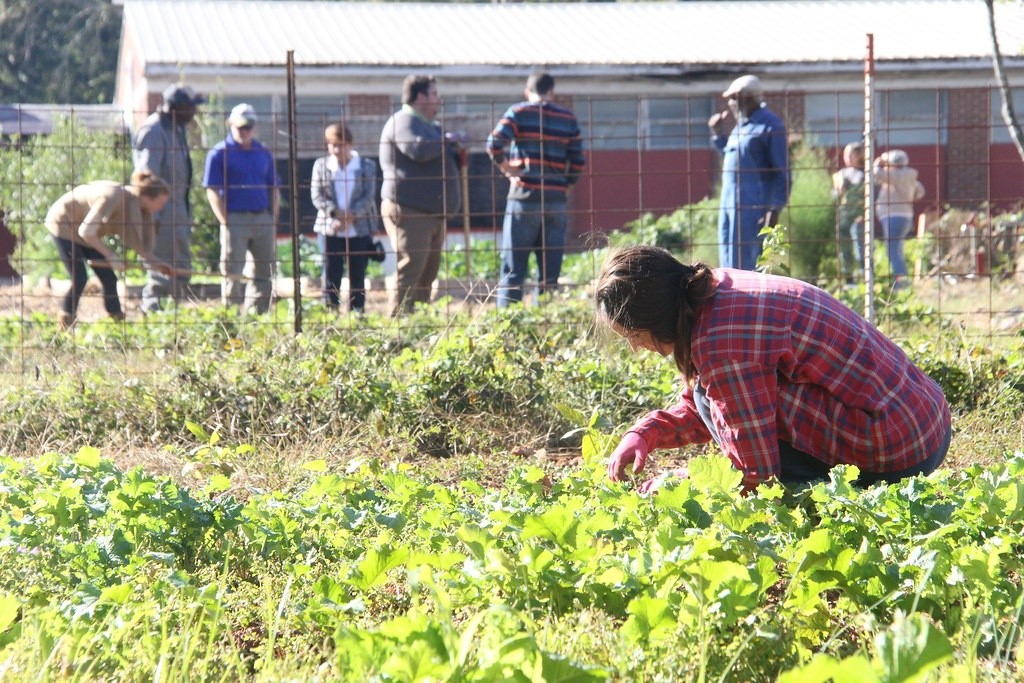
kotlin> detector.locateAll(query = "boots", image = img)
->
[56,314,77,332]
[109,312,125,323]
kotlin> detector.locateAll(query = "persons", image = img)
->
[873,149,925,290]
[709,75,791,271]
[486,73,586,311]
[202,103,280,317]
[595,247,953,494]
[132,83,196,314]
[378,75,470,316]
[46,172,173,331]
[307,124,379,314]
[832,142,877,288]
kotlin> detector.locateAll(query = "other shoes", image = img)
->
[841,284,856,291]
[890,280,908,291]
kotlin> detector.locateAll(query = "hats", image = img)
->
[881,149,908,166]
[163,83,204,105]
[228,104,256,129]
[722,75,762,105]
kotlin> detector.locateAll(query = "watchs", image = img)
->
[330,209,338,217]
